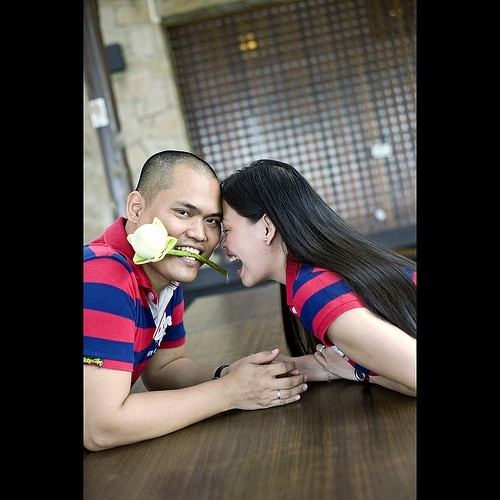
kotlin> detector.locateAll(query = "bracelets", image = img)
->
[323,367,331,381]
[212,364,229,380]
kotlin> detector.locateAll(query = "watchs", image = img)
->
[354,369,369,382]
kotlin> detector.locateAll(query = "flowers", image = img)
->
[127,217,229,277]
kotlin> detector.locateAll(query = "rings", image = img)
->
[320,346,326,352]
[277,389,280,399]
[321,360,325,366]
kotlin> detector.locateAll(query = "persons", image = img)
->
[220,160,415,398]
[83,149,308,453]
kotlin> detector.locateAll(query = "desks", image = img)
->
[83,281,416,500]
